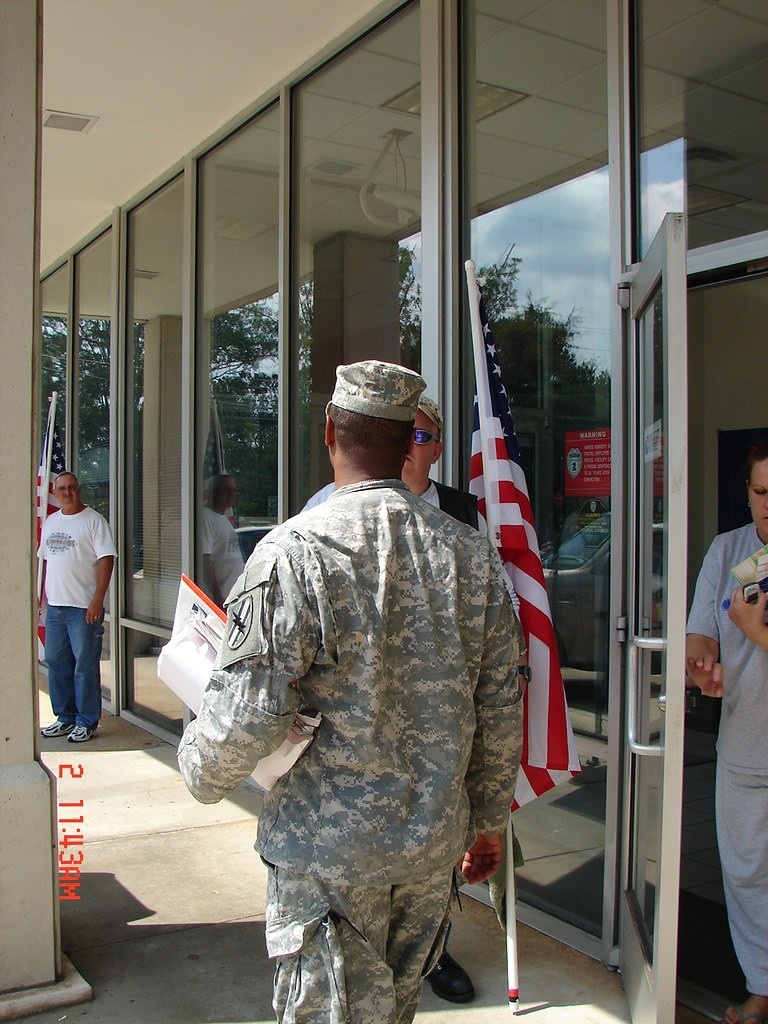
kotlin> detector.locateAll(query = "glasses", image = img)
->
[411,432,444,445]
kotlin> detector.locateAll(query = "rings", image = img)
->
[94,617,97,618]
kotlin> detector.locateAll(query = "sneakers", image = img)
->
[67,725,99,742]
[41,720,76,737]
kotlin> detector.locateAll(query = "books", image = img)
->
[158,574,321,793]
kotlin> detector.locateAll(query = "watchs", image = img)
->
[518,665,532,683]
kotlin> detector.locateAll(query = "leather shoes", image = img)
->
[423,952,475,1003]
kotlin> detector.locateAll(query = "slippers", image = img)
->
[722,1006,767,1024]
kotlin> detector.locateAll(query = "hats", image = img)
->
[418,397,444,431]
[330,359,429,421]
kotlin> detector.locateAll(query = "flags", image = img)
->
[204,416,233,523]
[469,299,580,813]
[38,406,66,663]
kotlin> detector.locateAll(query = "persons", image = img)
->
[36,472,117,742]
[200,476,245,602]
[686,441,768,1024]
[178,360,526,1023]
[299,397,532,1004]
